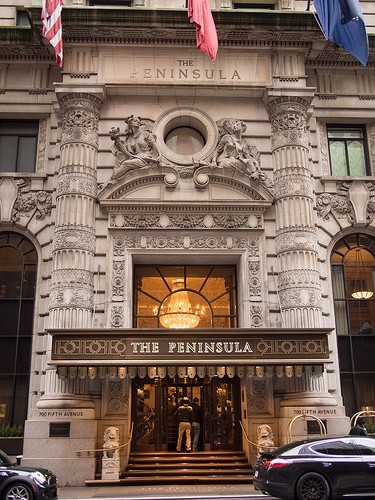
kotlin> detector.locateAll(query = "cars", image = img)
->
[252,433,375,500]
[0,447,59,500]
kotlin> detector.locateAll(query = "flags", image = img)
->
[312,0,369,67]
[41,0,64,71]
[186,0,219,64]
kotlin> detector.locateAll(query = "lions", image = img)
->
[103,427,120,459]
[257,425,274,457]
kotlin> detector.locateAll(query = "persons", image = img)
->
[349,417,367,437]
[108,114,162,180]
[210,117,262,181]
[174,397,193,453]
[189,397,202,451]
[168,393,176,415]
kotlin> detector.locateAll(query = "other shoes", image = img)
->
[193,448,198,451]
[186,450,190,453]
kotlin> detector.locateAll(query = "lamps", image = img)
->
[152,277,206,329]
[351,249,373,300]
